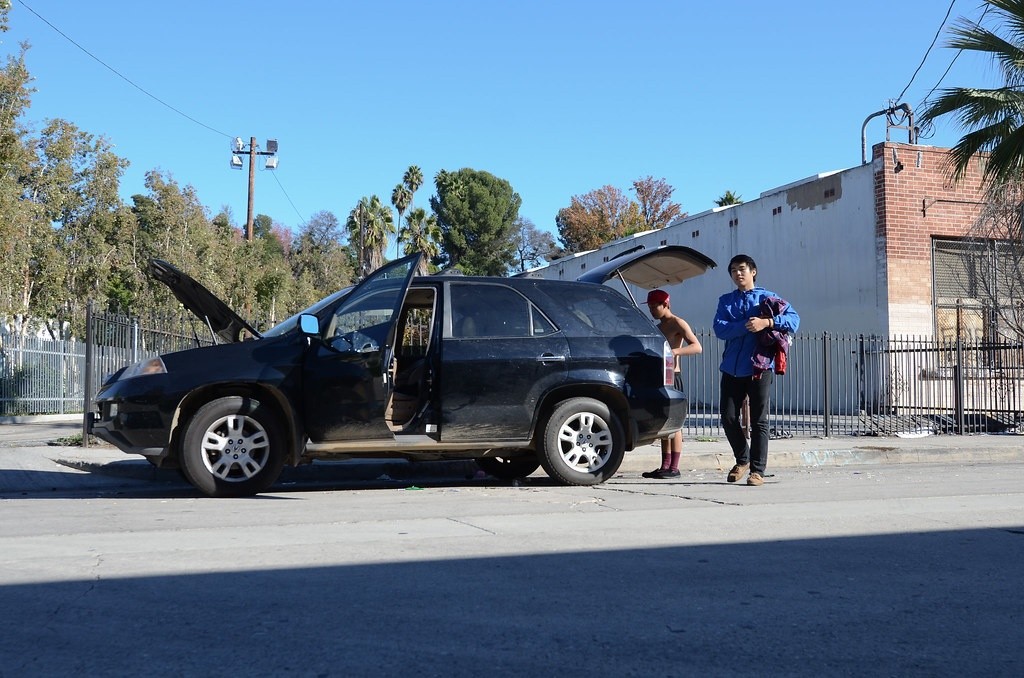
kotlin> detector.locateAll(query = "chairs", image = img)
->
[460,314,485,336]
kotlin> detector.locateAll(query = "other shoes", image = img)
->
[642,469,681,478]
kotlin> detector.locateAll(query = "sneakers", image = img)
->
[747,472,763,486]
[727,463,750,482]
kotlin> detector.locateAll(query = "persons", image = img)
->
[641,289,703,479]
[712,254,800,485]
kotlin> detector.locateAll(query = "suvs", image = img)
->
[88,244,718,493]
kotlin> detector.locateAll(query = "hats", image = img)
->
[641,290,670,305]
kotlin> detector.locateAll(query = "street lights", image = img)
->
[230,136,280,337]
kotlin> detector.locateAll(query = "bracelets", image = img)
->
[768,318,771,327]
